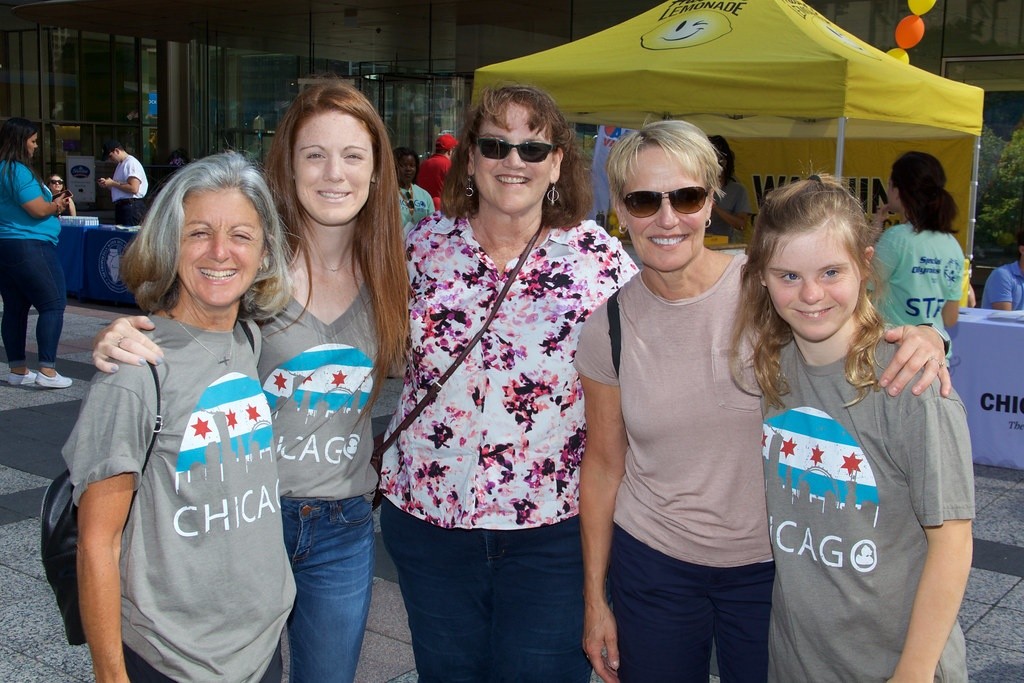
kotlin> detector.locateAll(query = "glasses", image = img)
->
[405,192,415,209]
[51,179,63,185]
[620,186,708,219]
[476,137,556,163]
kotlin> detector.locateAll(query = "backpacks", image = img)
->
[38,317,263,645]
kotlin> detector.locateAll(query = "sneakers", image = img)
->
[35,371,73,388]
[8,371,38,385]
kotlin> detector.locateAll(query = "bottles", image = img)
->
[596,210,604,228]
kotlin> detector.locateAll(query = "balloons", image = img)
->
[886,48,910,65]
[895,15,925,49]
[907,0,936,16]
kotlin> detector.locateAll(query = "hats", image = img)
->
[434,133,460,150]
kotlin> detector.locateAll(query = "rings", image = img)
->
[116,336,126,347]
[932,357,945,371]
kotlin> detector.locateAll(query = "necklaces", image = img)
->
[398,183,415,218]
[164,307,235,370]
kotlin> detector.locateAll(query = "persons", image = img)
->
[981,233,1024,310]
[392,147,435,230]
[706,133,751,244]
[97,141,148,225]
[92,79,405,683]
[62,149,298,683]
[377,84,640,683]
[729,173,975,683]
[417,134,459,199]
[573,119,952,683]
[866,152,965,372]
[46,173,76,218]
[0,117,73,389]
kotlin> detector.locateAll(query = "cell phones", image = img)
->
[52,190,73,202]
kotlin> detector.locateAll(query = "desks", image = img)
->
[944,309,1024,472]
[53,224,142,304]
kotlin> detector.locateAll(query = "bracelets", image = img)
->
[53,199,62,218]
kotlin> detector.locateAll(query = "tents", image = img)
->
[473,0,985,307]
